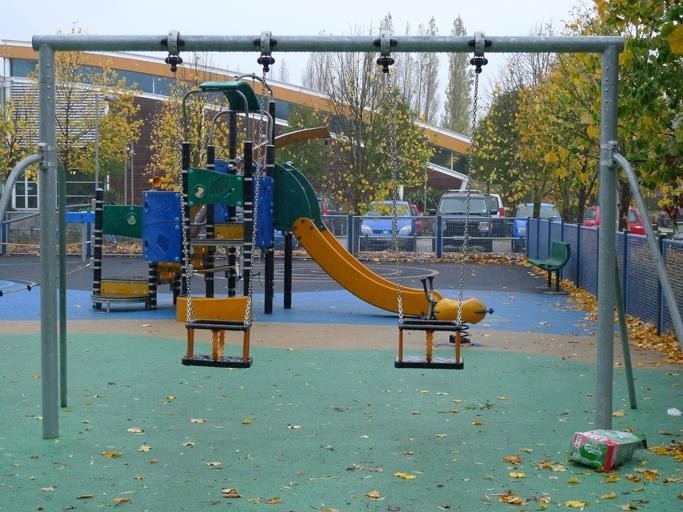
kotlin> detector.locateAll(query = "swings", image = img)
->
[161,31,278,368]
[373,31,491,370]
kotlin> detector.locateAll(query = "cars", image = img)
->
[431,192,505,252]
[360,201,423,252]
[511,203,561,252]
[583,205,653,236]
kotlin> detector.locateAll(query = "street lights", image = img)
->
[95,88,120,199]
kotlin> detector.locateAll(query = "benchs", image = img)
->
[527,241,571,294]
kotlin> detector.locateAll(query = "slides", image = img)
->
[273,163,442,317]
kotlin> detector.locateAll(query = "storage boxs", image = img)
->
[569,430,647,472]
